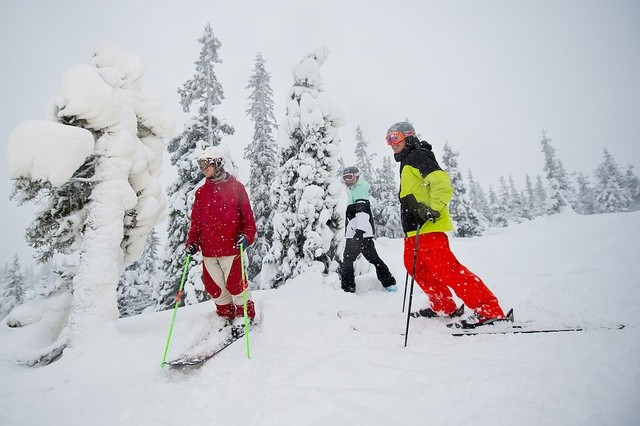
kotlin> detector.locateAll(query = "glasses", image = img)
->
[199,160,211,169]
[342,173,355,182]
[385,132,404,145]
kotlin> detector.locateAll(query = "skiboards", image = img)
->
[164,319,257,365]
[336,310,583,335]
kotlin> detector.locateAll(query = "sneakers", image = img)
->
[456,313,505,327]
[419,307,444,318]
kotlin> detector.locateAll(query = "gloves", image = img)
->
[186,243,196,257]
[419,207,440,221]
[353,230,365,248]
[237,236,249,250]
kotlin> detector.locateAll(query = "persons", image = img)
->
[184,146,257,337]
[341,166,397,292]
[385,122,506,331]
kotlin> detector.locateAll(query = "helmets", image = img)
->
[387,122,415,139]
[196,147,225,171]
[342,167,359,179]
